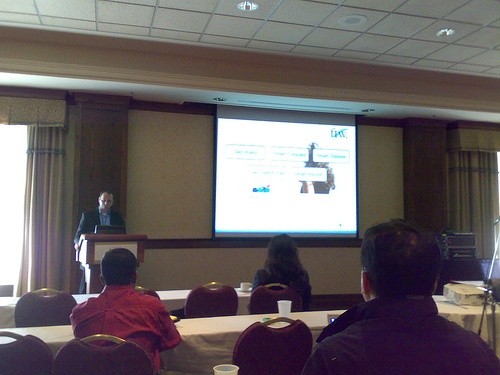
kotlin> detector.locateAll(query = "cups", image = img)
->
[213,364,239,375]
[277,300,292,318]
[241,282,250,291]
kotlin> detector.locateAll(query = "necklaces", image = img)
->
[102,212,107,225]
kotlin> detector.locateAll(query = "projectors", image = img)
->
[443,284,485,307]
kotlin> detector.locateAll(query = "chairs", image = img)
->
[14,287,77,327]
[0,332,53,375]
[52,334,156,375]
[185,282,238,318]
[249,283,303,313]
[233,317,313,375]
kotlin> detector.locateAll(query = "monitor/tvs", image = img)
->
[94,225,124,233]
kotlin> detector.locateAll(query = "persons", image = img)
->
[252,234,312,312]
[301,218,500,375]
[74,190,127,294]
[70,247,183,375]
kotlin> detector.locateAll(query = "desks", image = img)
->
[0,288,499,375]
